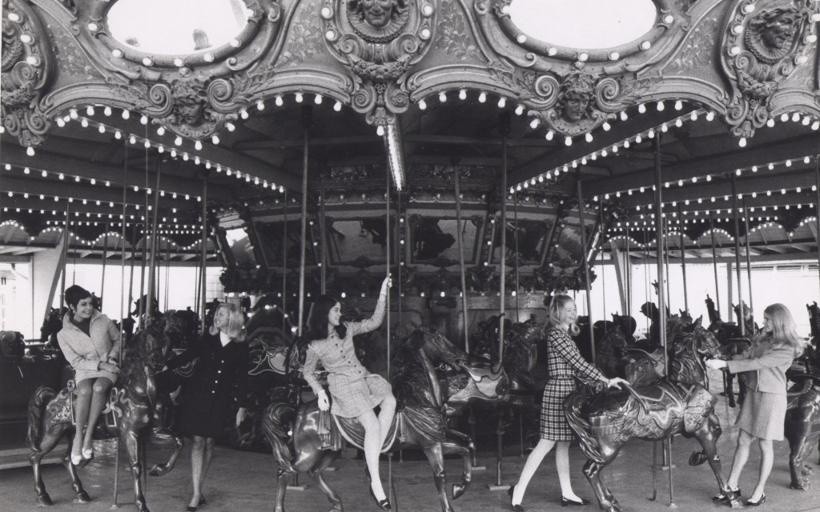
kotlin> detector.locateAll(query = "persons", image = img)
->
[507,294,630,512]
[302,272,397,510]
[56,285,121,466]
[702,304,802,505]
[183,302,248,511]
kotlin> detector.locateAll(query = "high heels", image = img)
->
[713,490,766,506]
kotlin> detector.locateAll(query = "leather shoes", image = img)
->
[370,485,391,511]
[561,497,589,506]
[71,448,92,465]
[509,485,523,512]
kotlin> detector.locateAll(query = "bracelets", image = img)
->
[606,378,611,386]
